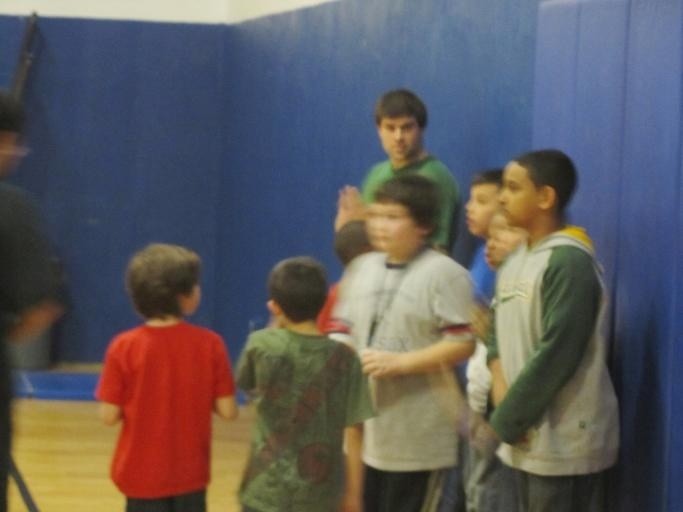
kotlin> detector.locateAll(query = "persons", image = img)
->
[311,216,382,339]
[89,241,241,511]
[225,251,380,510]
[464,207,533,422]
[329,86,468,272]
[454,165,523,286]
[1,89,78,512]
[466,146,625,511]
[309,172,486,511]
